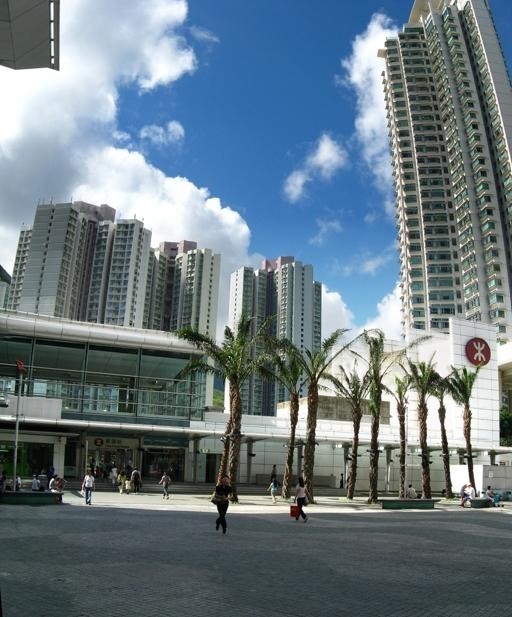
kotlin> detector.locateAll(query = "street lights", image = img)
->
[11,363,25,489]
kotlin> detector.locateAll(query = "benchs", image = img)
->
[470,499,493,508]
[376,499,441,509]
[0,490,65,504]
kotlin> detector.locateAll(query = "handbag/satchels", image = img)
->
[303,495,310,507]
[290,505,300,518]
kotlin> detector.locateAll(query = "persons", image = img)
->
[0,459,142,505]
[294,477,312,523]
[267,479,279,504]
[407,484,418,498]
[458,484,497,508]
[157,471,172,500]
[215,474,233,537]
[270,464,277,483]
